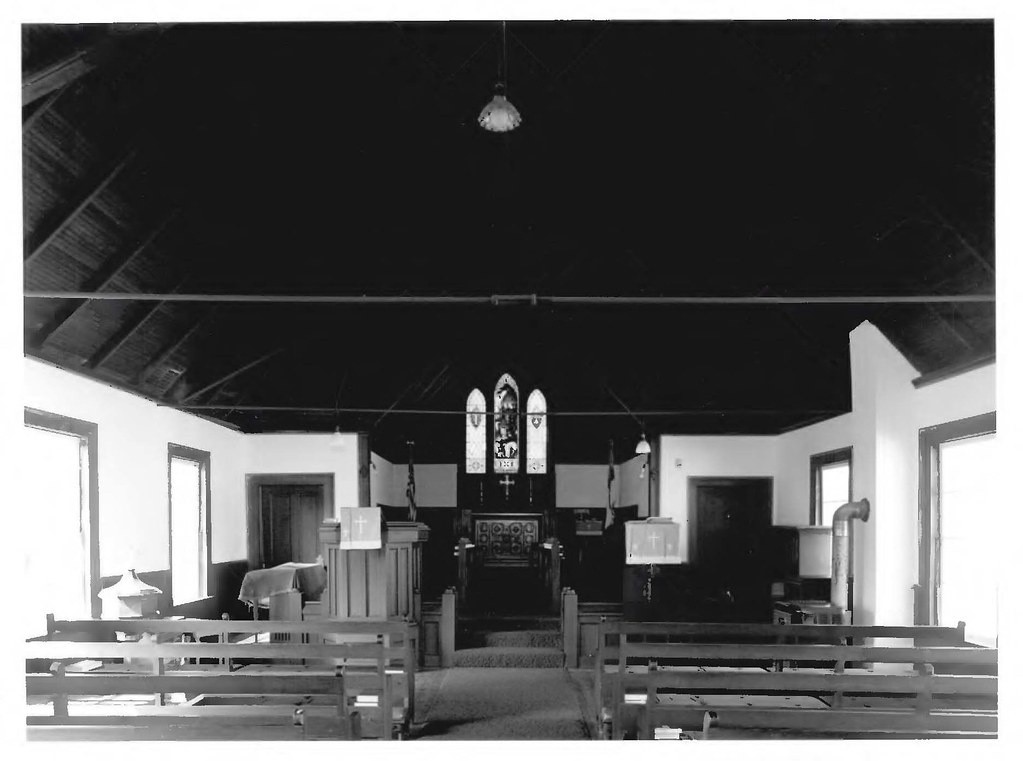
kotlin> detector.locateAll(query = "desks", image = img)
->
[238,560,327,643]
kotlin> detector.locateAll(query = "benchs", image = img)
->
[592,613,998,740]
[25,612,420,741]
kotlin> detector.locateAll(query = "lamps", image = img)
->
[635,433,652,454]
[478,21,521,132]
